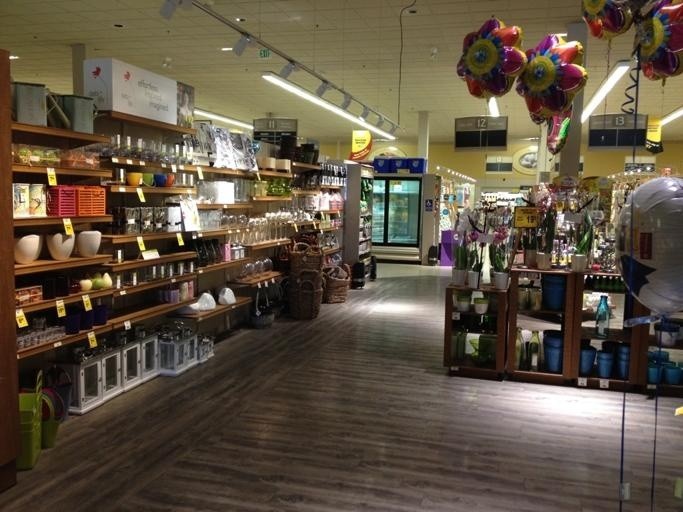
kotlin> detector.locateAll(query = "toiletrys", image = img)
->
[158,279,194,304]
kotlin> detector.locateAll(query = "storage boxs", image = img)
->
[81,56,178,126]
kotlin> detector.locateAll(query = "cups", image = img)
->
[110,260,194,290]
[473,298,490,314]
[543,332,683,386]
[15,318,68,350]
[157,281,194,303]
[102,135,195,165]
[126,172,172,187]
[197,210,291,244]
[467,270,479,289]
[111,206,125,236]
[13,229,102,265]
[456,295,470,311]
[229,178,268,202]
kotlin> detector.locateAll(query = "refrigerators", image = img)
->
[372,177,421,264]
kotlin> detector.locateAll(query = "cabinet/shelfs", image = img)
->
[433,263,681,399]
[10,117,377,416]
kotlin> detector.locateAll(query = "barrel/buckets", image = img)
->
[541,329,680,385]
[329,193,337,210]
[542,272,568,313]
[336,192,344,210]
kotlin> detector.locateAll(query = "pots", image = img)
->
[103,167,126,185]
[166,173,193,187]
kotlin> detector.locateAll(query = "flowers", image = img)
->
[443,194,596,291]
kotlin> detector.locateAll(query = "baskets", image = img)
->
[46,184,77,219]
[325,261,352,303]
[75,184,106,218]
[282,280,325,321]
[289,240,326,272]
[287,266,324,290]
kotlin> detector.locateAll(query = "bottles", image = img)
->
[595,294,609,337]
[231,243,245,260]
[523,288,531,309]
[535,288,542,306]
[455,326,469,360]
[526,330,541,370]
[513,327,524,369]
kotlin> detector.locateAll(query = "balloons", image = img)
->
[456,16,527,97]
[631,0,683,80]
[579,0,660,39]
[516,31,587,123]
[615,177,683,315]
